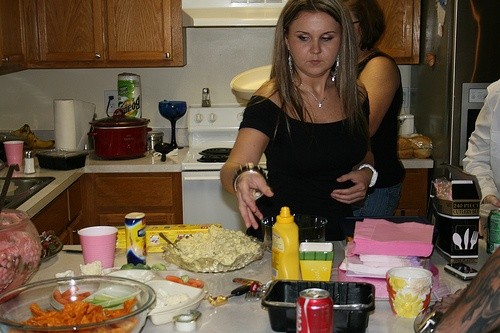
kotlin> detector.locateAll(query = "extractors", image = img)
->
[181,0,288,27]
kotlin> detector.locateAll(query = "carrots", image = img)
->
[165,275,203,288]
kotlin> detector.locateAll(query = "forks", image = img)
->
[470,231,479,249]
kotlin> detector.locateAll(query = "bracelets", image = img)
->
[233,163,267,200]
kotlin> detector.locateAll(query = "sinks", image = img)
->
[0,176,56,214]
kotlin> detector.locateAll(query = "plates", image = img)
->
[161,235,265,272]
[40,238,62,262]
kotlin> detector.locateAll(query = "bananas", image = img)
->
[17,125,55,148]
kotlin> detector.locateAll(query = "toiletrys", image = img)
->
[24,151,36,173]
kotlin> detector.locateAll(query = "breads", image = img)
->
[398,133,433,158]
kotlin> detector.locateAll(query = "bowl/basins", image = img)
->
[104,268,206,324]
[35,148,88,169]
[261,280,375,333]
[0,276,156,333]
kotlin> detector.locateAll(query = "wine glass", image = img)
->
[158,101,187,153]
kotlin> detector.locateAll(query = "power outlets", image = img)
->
[402,87,409,108]
[104,90,118,113]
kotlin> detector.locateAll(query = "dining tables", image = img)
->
[0,240,492,333]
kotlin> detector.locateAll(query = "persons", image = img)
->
[221,0,407,242]
[460,77,500,240]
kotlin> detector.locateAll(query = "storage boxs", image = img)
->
[432,174,482,264]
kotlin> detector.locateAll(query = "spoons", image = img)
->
[453,232,463,250]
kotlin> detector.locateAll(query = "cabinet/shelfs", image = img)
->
[30,172,183,247]
[374,0,420,65]
[394,169,427,222]
[0,0,186,76]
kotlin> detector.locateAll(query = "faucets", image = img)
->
[0,162,20,214]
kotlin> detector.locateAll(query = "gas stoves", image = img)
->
[180,104,268,170]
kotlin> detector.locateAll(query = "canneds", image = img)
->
[296,288,333,333]
[485,208,500,255]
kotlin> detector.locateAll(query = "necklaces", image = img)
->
[295,81,327,108]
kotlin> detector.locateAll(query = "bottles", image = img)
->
[201,87,212,107]
[125,213,146,264]
[270,208,299,280]
[23,150,35,173]
[117,73,143,118]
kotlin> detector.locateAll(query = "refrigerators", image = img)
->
[408,0,500,169]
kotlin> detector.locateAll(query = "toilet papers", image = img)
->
[53,99,96,151]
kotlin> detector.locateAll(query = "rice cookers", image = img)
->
[89,108,154,159]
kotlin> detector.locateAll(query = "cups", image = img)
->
[387,266,434,319]
[3,140,24,171]
[78,226,117,271]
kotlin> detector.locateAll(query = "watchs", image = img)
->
[358,164,378,187]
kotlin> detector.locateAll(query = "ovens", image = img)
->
[181,170,247,235]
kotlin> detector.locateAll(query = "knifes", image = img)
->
[464,228,470,250]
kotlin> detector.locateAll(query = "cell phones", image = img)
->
[444,262,479,281]
